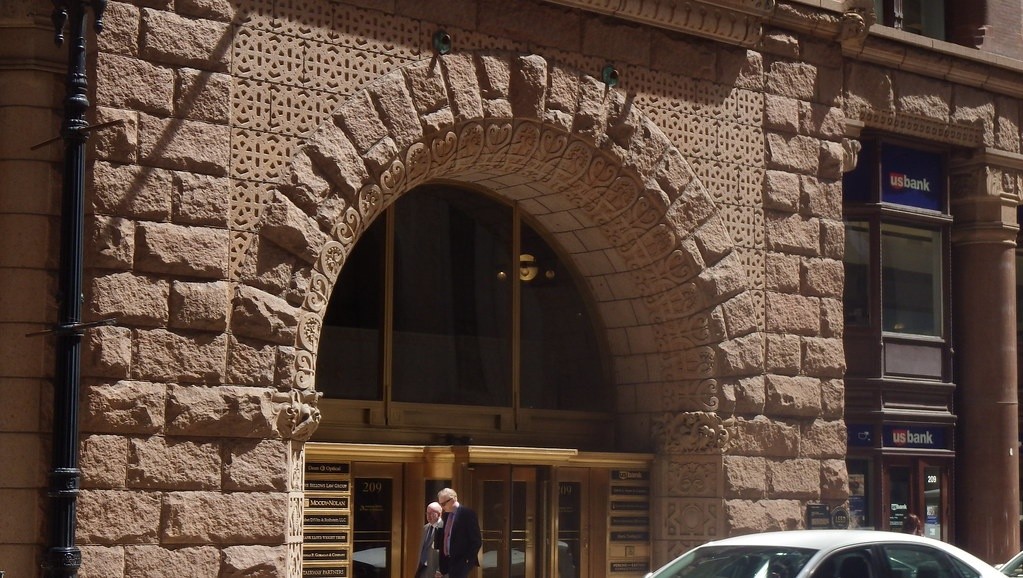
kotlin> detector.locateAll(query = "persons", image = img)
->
[438,487,482,578]
[415,502,444,578]
[902,513,920,534]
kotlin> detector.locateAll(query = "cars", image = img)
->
[643,530,1023,578]
[482,540,567,570]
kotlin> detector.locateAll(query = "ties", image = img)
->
[444,513,454,556]
[421,526,433,566]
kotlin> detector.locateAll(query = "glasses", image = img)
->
[440,498,453,507]
